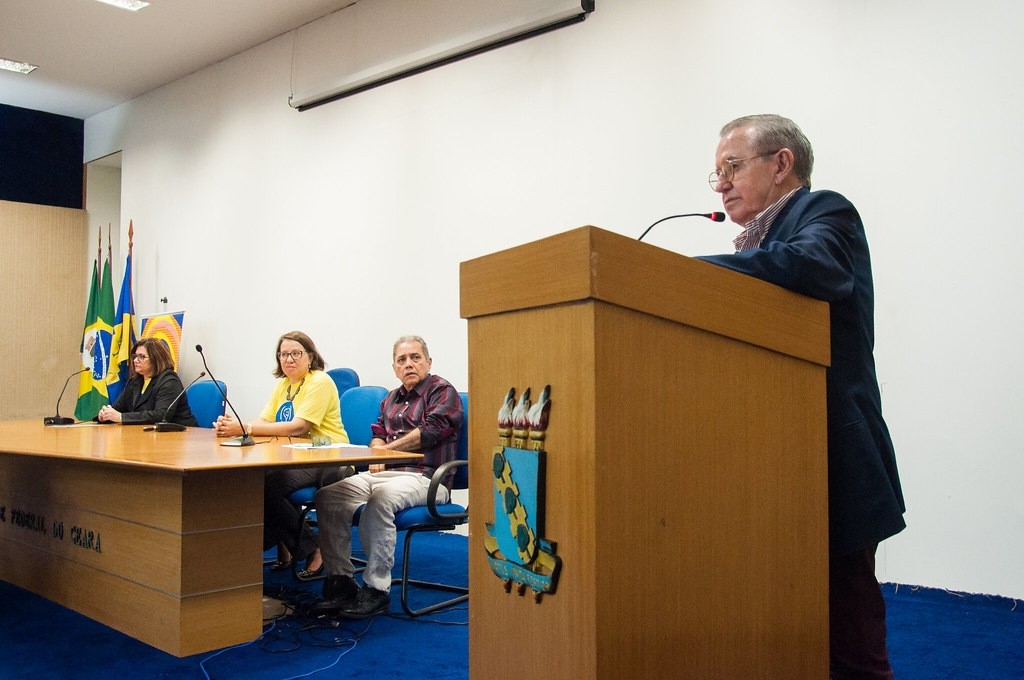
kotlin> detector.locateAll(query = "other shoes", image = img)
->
[296,562,327,581]
[271,554,294,570]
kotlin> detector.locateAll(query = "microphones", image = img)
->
[196,345,255,447]
[44,367,91,425]
[153,372,206,433]
[637,212,726,241]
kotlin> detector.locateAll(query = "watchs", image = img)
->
[246,424,252,436]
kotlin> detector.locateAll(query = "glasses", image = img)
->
[130,353,150,362]
[708,150,781,192]
[276,350,308,361]
[289,432,332,447]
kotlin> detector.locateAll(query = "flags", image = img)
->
[74,251,137,420]
[141,311,183,372]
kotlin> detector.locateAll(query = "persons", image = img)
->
[212,330,356,577]
[97,338,199,428]
[313,337,464,620]
[689,114,906,680]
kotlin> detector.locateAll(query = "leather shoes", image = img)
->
[337,584,391,618]
[317,575,362,610]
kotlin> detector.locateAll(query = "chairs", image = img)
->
[186,380,227,428]
[288,369,469,617]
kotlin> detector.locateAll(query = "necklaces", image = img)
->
[286,377,305,401]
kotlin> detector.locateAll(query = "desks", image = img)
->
[0,419,424,658]
